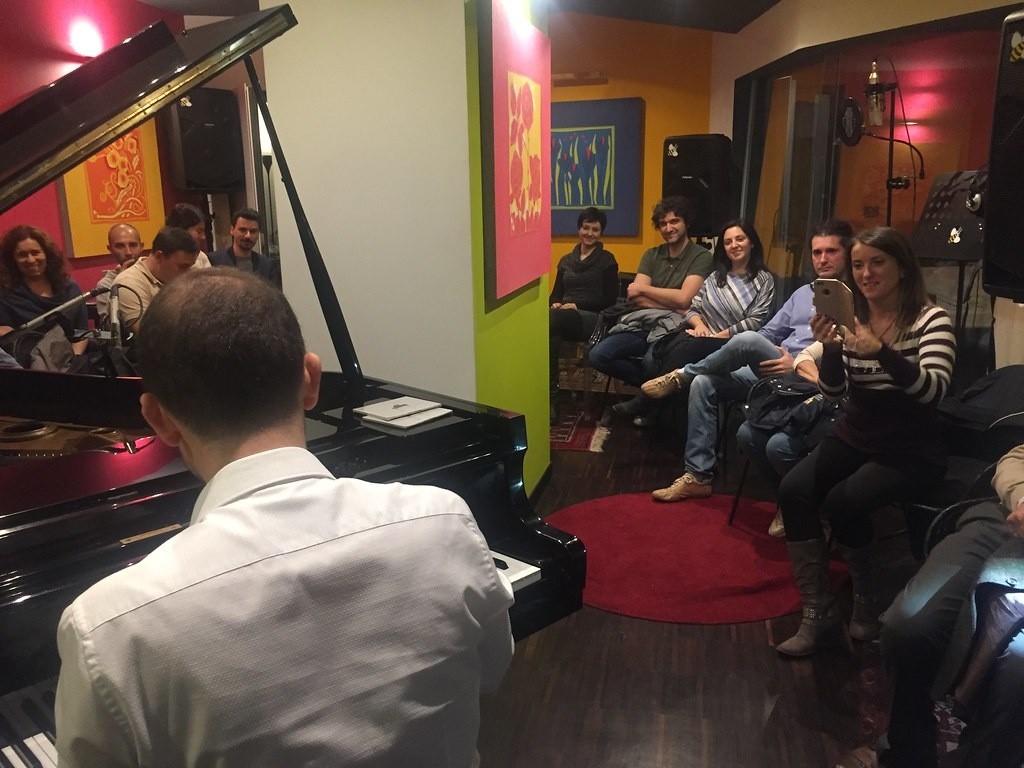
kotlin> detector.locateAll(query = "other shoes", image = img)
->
[612,394,652,420]
[550,387,560,426]
[767,506,787,540]
[633,413,653,425]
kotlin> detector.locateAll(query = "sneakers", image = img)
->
[652,472,712,502]
[640,369,684,399]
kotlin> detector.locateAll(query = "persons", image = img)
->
[549,200,1024,768]
[208,208,277,286]
[167,203,211,268]
[0,225,90,355]
[53,264,514,768]
[96,223,144,332]
[109,228,199,335]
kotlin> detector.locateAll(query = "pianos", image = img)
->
[0,2,588,768]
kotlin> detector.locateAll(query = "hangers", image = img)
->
[549,403,612,453]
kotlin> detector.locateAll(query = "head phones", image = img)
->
[964,170,987,213]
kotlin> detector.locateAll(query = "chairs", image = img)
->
[572,269,1002,564]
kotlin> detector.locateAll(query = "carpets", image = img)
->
[557,356,641,393]
[543,492,851,623]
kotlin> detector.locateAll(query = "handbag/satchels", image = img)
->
[743,371,820,430]
[587,301,634,350]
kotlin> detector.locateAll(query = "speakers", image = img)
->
[165,89,246,194]
[662,133,739,238]
[981,10,1024,304]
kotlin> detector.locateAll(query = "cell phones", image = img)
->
[813,279,855,337]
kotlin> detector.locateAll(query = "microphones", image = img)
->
[110,283,122,349]
[0,287,108,348]
[868,61,883,126]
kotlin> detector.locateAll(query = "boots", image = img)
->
[775,535,843,656]
[833,531,890,639]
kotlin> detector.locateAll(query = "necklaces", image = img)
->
[869,318,895,342]
